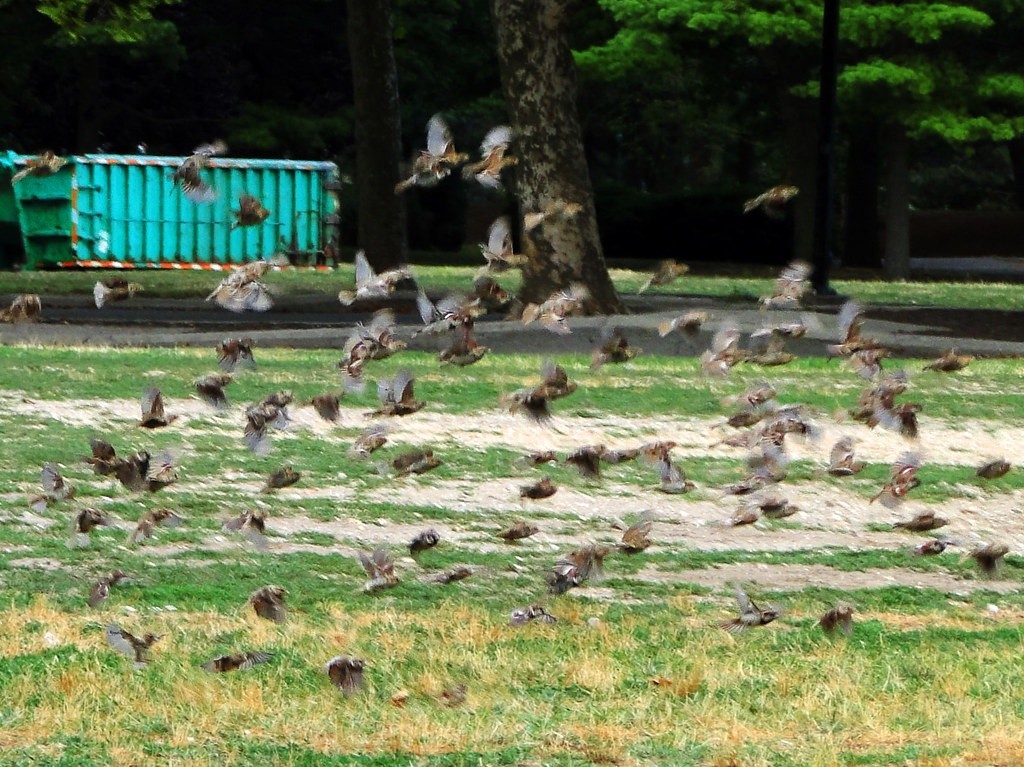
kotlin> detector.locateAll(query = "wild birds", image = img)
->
[0,114,1016,709]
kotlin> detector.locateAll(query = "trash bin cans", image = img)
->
[1,150,340,273]
